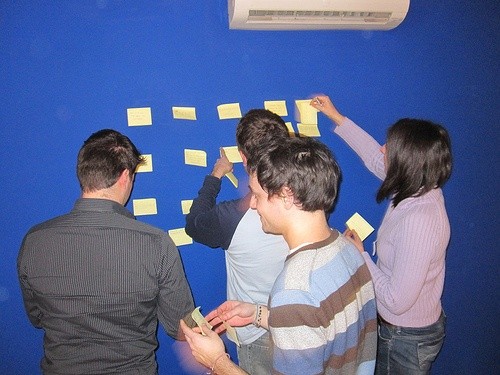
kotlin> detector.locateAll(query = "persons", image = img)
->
[185,132,378,374]
[184,109,290,375]
[309,96,452,375]
[16,129,195,375]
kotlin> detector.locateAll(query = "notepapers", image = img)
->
[345,212,375,243]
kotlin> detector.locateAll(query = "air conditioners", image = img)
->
[228,0,411,30]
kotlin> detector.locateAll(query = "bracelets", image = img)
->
[211,353,230,373]
[253,304,262,329]
